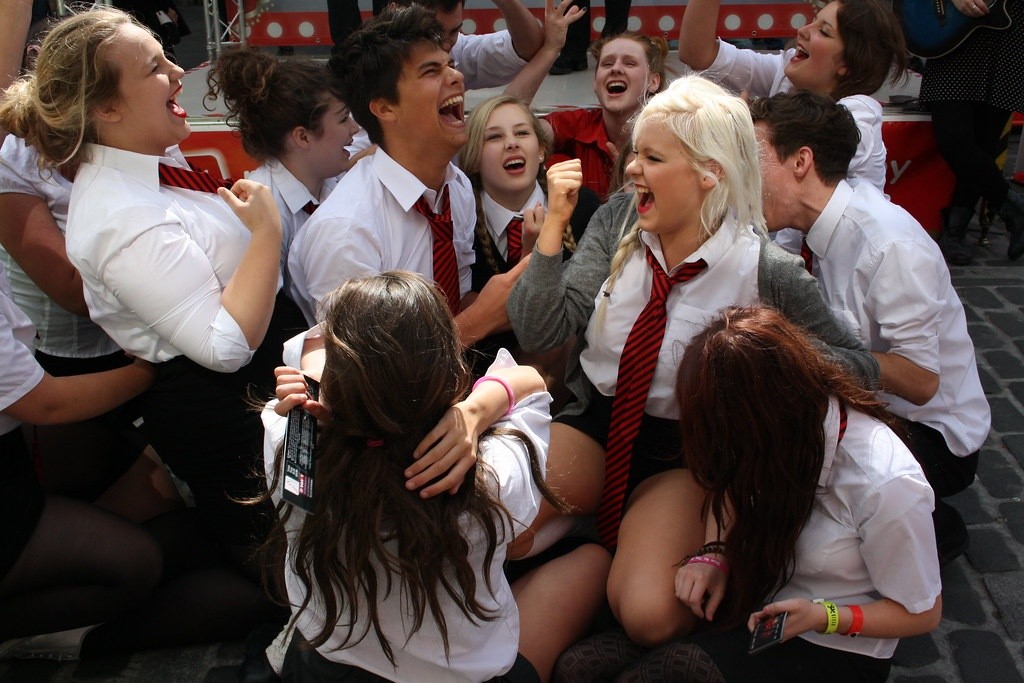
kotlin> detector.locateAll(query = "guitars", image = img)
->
[892,1,1015,62]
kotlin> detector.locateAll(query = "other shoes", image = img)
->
[940,509,968,569]
[0,622,105,662]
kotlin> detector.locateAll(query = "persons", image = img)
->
[678,0,887,272]
[509,70,882,645]
[0,1,578,683]
[553,308,943,683]
[752,88,990,549]
[919,0,1024,262]
[261,273,552,683]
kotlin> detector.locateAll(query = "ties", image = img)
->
[507,216,523,266]
[836,399,848,446]
[300,201,320,214]
[413,184,461,316]
[802,239,813,274]
[597,245,707,560]
[159,162,233,192]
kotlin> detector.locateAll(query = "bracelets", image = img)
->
[473,377,515,417]
[811,598,838,636]
[841,604,864,638]
[688,541,731,576]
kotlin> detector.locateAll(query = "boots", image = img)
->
[941,206,972,265]
[998,188,1024,260]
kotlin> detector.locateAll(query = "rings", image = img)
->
[972,5,976,10]
